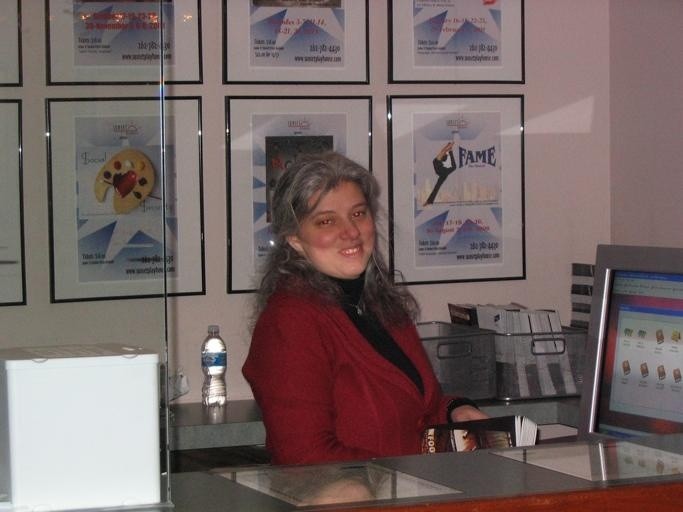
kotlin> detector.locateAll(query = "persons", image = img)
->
[423,143,457,208]
[240,151,490,466]
[262,457,402,507]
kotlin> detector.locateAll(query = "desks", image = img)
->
[162,434,683,512]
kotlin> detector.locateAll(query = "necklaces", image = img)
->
[345,291,365,316]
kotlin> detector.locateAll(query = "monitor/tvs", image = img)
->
[578,244,683,446]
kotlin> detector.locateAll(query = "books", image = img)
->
[448,301,577,399]
[420,414,538,454]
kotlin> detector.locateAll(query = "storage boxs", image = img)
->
[492,330,588,405]
[415,319,499,405]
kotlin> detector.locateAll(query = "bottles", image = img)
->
[199,324,228,409]
